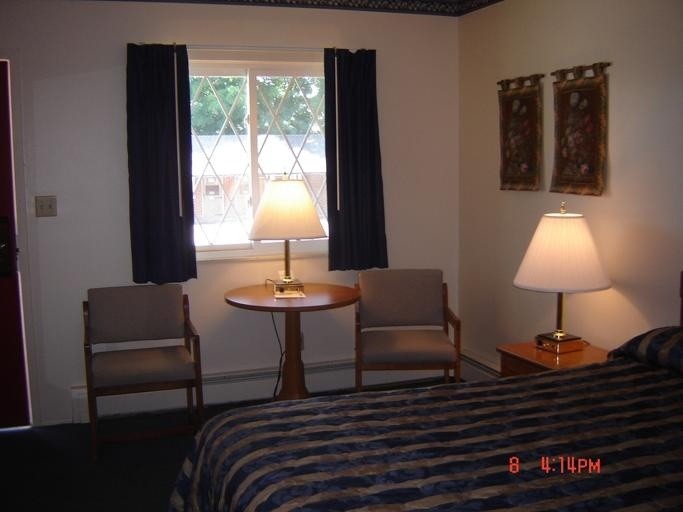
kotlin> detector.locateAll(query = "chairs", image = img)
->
[352,268,461,391]
[80,282,205,462]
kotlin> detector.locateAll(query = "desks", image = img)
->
[225,282,361,402]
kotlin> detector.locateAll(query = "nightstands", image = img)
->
[493,341,609,375]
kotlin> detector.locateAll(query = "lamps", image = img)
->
[512,200,613,357]
[246,171,327,293]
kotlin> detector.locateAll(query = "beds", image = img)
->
[186,326,682,511]
